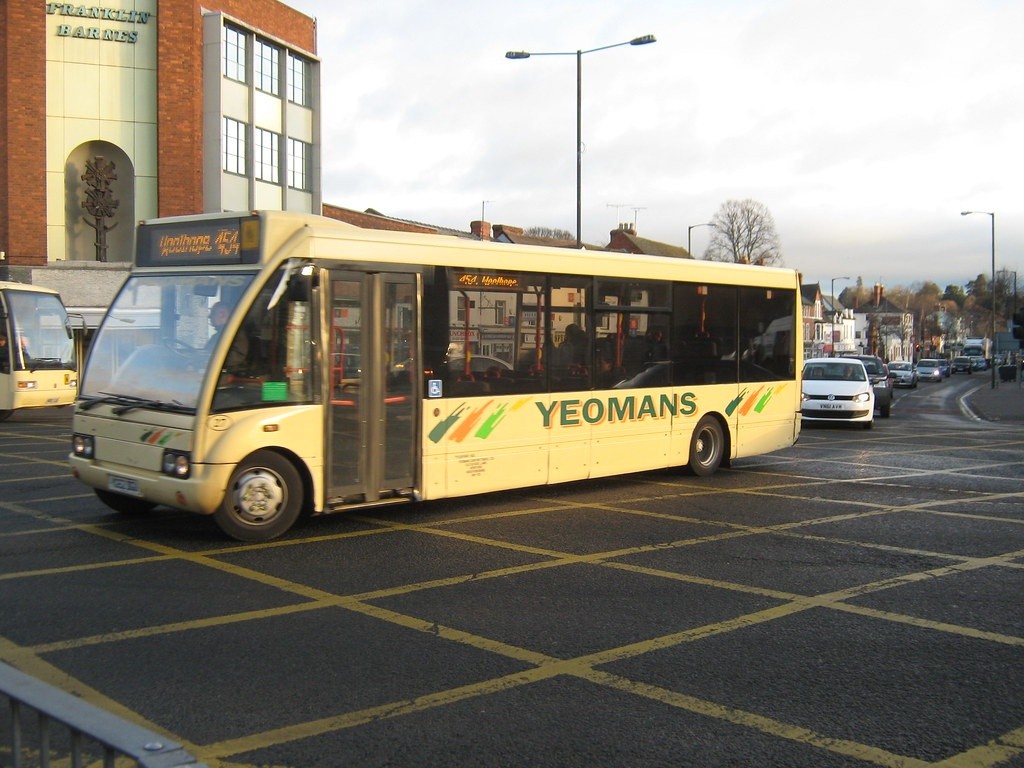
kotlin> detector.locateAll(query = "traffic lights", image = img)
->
[916,344,920,353]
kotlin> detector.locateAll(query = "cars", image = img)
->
[888,361,918,388]
[939,355,987,377]
[882,363,894,400]
[799,357,880,430]
[915,359,943,382]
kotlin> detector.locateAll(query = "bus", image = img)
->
[68,206,806,542]
[0,279,88,424]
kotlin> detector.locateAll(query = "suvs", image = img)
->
[845,355,897,418]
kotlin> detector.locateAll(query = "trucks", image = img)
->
[961,337,994,369]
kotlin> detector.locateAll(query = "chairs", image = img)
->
[868,365,876,374]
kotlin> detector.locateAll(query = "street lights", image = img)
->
[688,223,717,260]
[960,209,996,390]
[997,270,1017,335]
[831,277,852,357]
[504,33,658,250]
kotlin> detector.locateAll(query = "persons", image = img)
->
[183,301,249,376]
[557,324,752,391]
[0,334,30,374]
[1021,359,1024,381]
[813,367,824,377]
[841,366,856,380]
